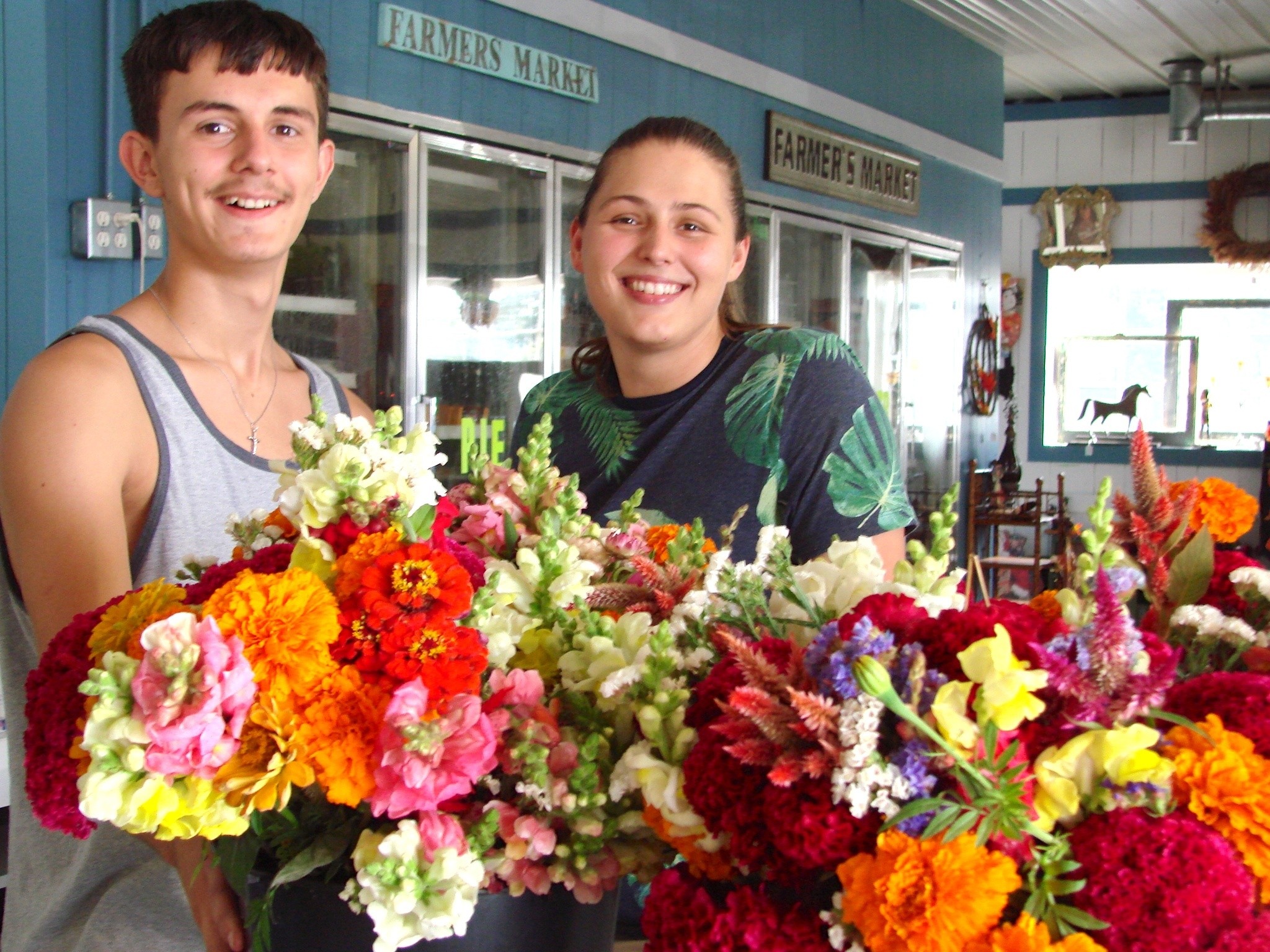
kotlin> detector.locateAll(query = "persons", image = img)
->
[512,115,920,951]
[1199,391,1212,439]
[0,0,377,952]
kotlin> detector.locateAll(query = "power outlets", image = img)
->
[69,197,164,260]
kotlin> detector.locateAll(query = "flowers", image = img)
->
[25,394,1270,952]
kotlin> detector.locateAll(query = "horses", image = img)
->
[1076,383,1153,432]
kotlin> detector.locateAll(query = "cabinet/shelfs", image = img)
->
[966,459,1065,600]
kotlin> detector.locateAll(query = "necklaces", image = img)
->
[147,286,276,456]
